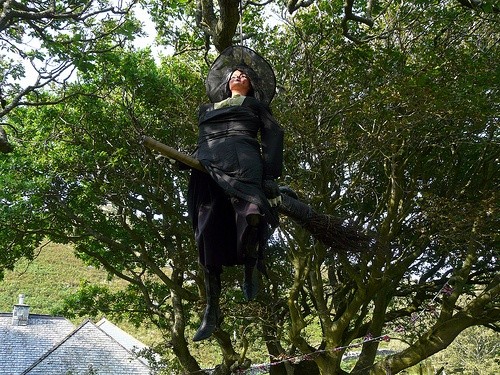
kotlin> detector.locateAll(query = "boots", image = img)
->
[244,224,260,302]
[193,269,222,341]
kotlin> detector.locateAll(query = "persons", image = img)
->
[186,45,300,342]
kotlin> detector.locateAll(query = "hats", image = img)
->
[206,46,276,105]
